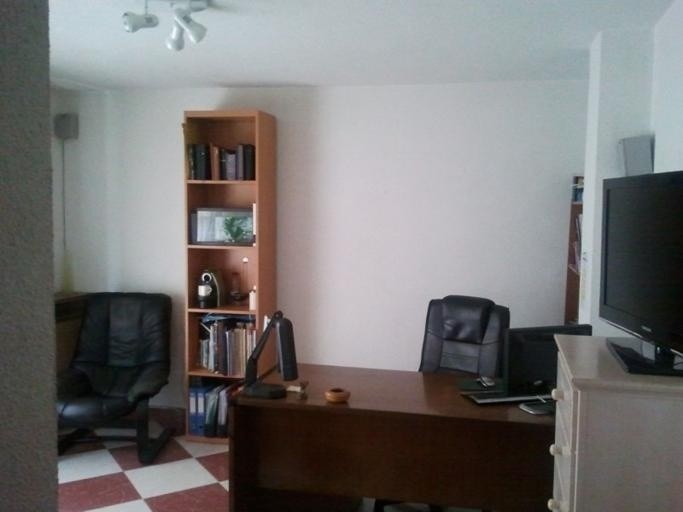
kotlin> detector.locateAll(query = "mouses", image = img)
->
[475,375,496,387]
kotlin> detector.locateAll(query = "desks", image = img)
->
[228,362,556,512]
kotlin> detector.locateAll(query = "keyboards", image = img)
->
[462,389,552,404]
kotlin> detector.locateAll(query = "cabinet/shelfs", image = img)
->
[563,176,584,327]
[182,109,276,444]
[546,334,682,512]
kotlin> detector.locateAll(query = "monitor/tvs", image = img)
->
[598,170,682,377]
[503,324,593,415]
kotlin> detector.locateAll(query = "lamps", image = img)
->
[121,0,158,32]
[164,11,191,51]
[173,5,207,45]
[240,311,298,400]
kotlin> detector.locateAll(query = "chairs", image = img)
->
[416,294,510,379]
[54,292,176,465]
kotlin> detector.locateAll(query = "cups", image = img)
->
[198,286,213,298]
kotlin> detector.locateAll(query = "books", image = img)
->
[197,318,256,377]
[191,207,253,245]
[189,385,241,436]
[572,210,582,274]
[185,140,255,182]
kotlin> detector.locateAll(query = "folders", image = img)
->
[198,386,207,435]
[188,386,197,435]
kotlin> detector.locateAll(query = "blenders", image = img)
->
[196,251,225,310]
